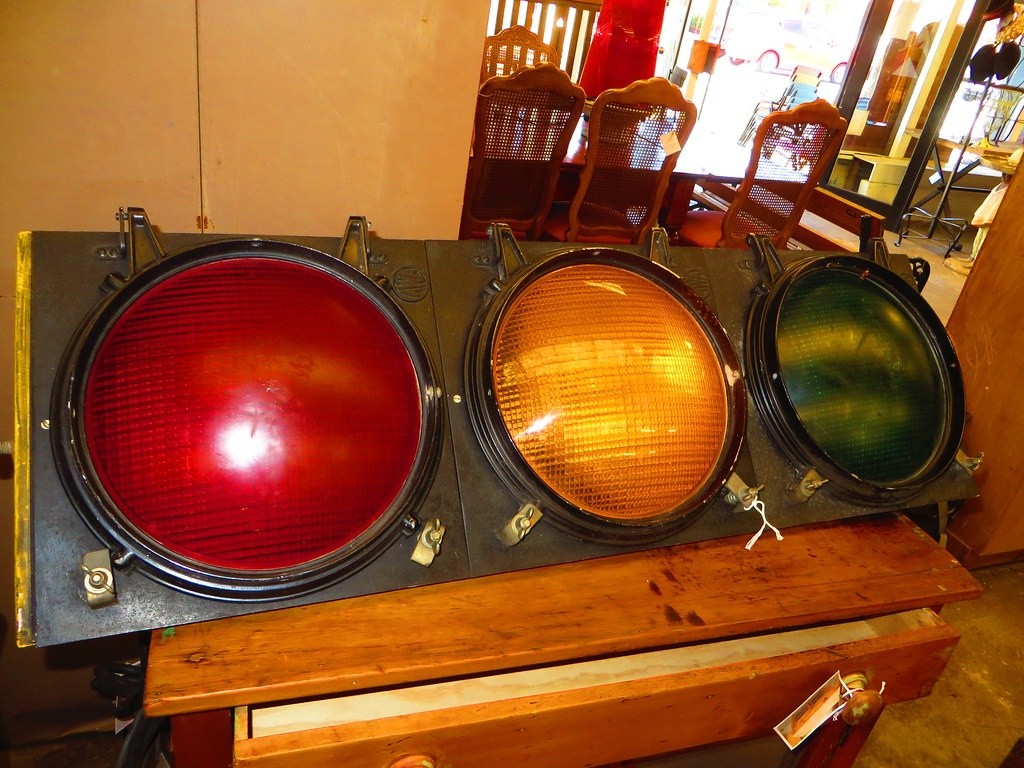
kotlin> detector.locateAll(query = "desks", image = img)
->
[144,514,985,768]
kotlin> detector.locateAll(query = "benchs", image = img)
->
[689,180,932,295]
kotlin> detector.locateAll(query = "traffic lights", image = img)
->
[14,211,981,662]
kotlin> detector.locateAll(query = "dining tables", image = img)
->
[470,104,820,246]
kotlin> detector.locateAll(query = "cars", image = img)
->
[724,8,855,82]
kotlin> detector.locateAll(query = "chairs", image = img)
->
[542,77,698,244]
[457,61,586,241]
[737,67,828,173]
[675,99,849,248]
[479,26,559,82]
